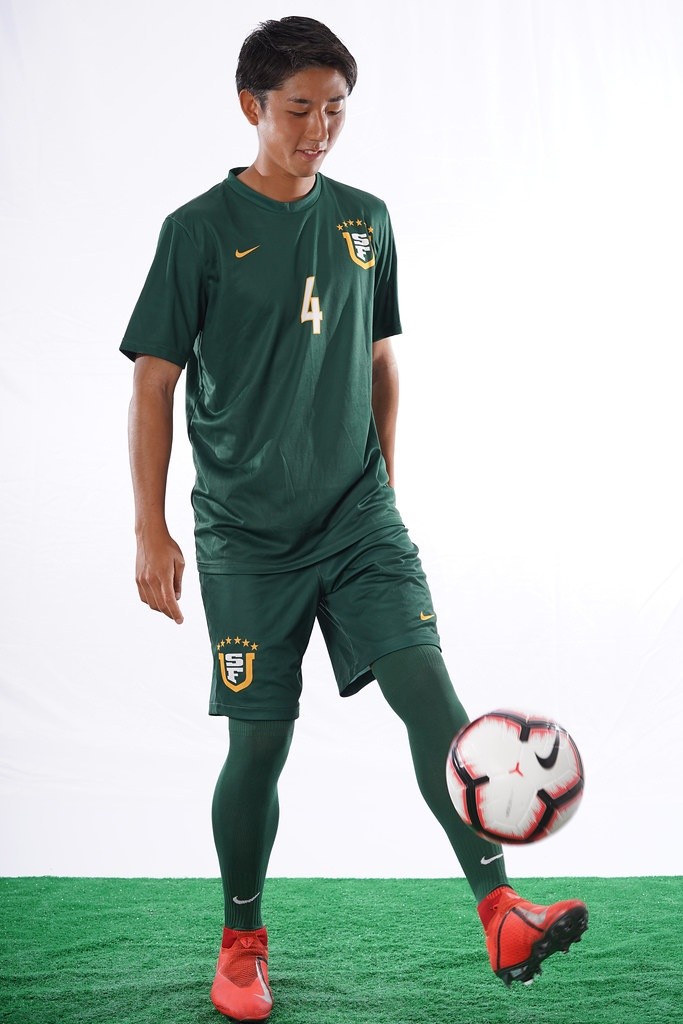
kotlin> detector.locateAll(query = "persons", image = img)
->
[120,13,591,1024]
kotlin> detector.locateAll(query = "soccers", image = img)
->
[446,709,583,846]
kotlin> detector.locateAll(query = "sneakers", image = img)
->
[209,930,272,1022]
[484,890,588,990]
